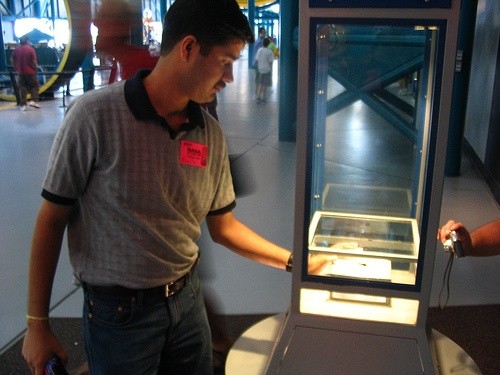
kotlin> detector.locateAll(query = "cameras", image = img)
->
[443,229,463,258]
[45,355,69,375]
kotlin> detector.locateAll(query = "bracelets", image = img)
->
[24,314,50,321]
[286,252,293,273]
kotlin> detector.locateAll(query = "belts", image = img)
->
[83,251,201,299]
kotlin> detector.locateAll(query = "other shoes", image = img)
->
[28,100,40,108]
[19,105,27,111]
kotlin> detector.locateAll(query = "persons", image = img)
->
[255,37,273,103]
[23,0,293,375]
[251,27,267,99]
[268,36,275,52]
[437,219,500,258]
[13,35,41,111]
